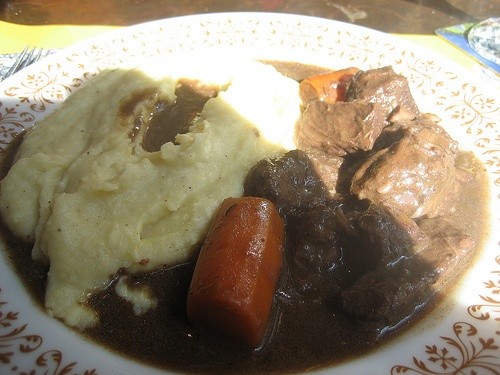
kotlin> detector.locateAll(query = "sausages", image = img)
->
[299,67,364,105]
[185,196,284,355]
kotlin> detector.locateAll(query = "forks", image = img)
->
[1,42,51,81]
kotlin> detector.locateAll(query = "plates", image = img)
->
[468,17,500,64]
[0,13,500,375]
